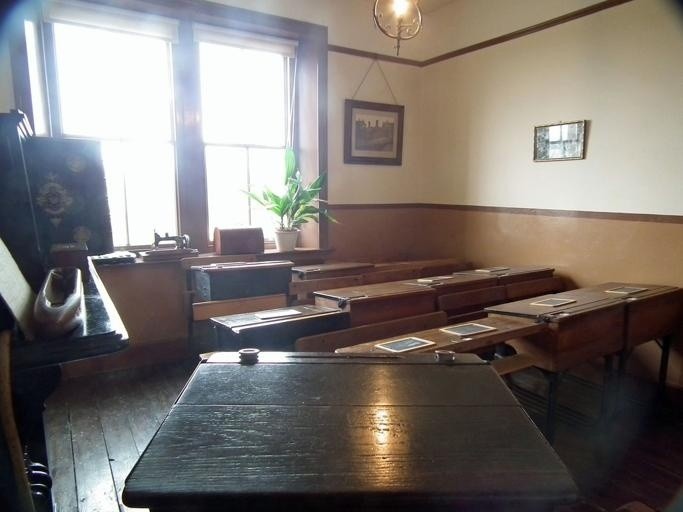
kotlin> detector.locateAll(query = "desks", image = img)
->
[189,258,683,446]
[121,348,579,512]
[0,256,131,511]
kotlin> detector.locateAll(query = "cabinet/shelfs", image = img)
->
[0,108,115,284]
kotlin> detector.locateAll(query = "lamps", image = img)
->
[373,0,422,56]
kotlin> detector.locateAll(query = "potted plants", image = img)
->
[241,146,341,251]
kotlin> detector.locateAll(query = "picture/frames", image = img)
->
[533,120,586,162]
[344,99,405,166]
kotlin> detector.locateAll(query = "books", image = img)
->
[474,265,509,273]
[528,298,576,308]
[373,336,437,354]
[604,285,649,294]
[255,308,302,319]
[438,323,496,336]
[416,276,455,282]
